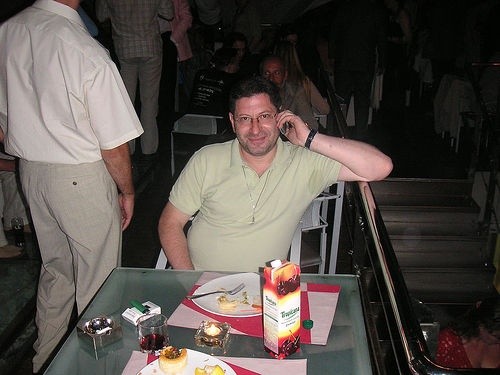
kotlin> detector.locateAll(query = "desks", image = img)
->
[42,267,372,375]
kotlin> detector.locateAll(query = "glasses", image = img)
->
[233,110,278,125]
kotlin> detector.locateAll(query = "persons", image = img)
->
[96,0,500,178]
[158,76,394,292]
[0,0,144,375]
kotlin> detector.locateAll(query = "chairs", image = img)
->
[155,215,302,269]
[170,115,223,177]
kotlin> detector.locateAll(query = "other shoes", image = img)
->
[24,232,41,259]
[5,231,16,245]
[143,151,159,161]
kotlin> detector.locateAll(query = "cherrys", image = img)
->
[277,268,301,296]
[265,330,300,357]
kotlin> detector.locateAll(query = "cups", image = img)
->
[137,313,170,356]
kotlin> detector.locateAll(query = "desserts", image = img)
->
[218,297,231,309]
[159,346,187,375]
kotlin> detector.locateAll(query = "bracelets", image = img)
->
[305,129,319,150]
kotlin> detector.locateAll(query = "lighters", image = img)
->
[131,300,151,316]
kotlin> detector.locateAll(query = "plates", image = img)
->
[191,272,264,318]
[136,349,238,375]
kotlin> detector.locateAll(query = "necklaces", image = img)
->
[242,166,270,222]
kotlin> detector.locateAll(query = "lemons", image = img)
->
[195,365,225,375]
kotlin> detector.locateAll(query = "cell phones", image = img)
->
[278,106,289,136]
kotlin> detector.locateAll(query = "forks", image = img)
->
[185,283,245,300]
